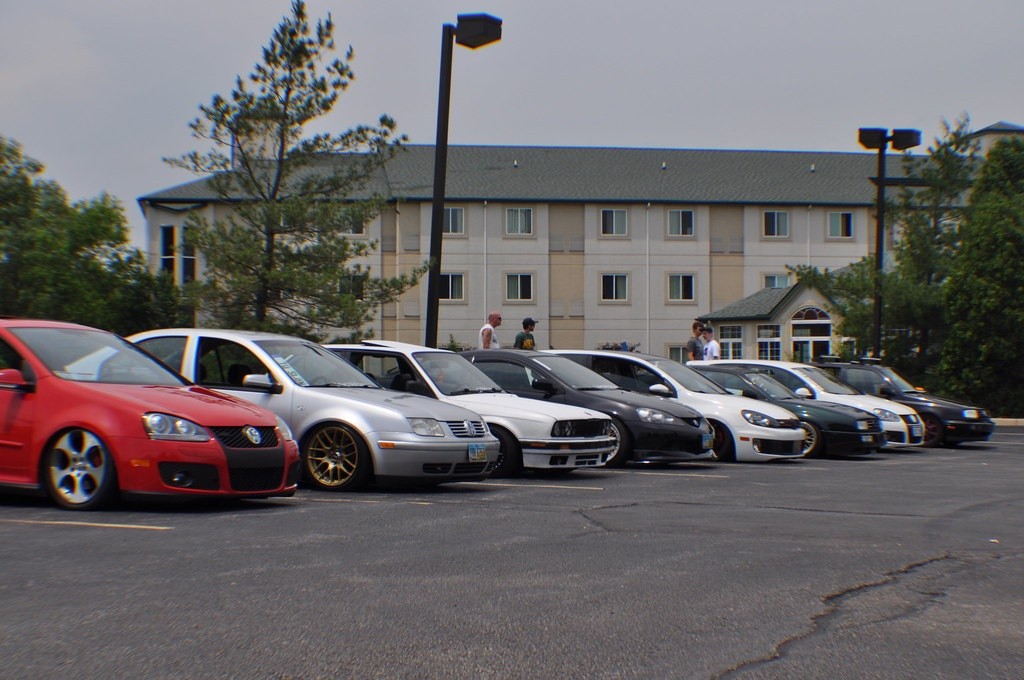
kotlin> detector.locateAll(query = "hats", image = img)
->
[699,328,712,334]
[521,318,538,326]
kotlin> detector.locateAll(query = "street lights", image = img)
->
[425,10,503,350]
[855,125,923,361]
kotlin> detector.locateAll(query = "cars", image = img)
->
[0,316,301,512]
[684,356,927,451]
[54,325,500,494]
[375,347,720,470]
[809,362,996,448]
[283,338,618,481]
[524,347,806,465]
[637,364,890,459]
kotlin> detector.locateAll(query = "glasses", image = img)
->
[494,317,502,321]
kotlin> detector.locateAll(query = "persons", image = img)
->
[687,322,704,361]
[479,311,502,349]
[514,318,538,350]
[700,328,720,361]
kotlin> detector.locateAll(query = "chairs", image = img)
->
[390,373,413,391]
[227,364,251,387]
[199,363,206,385]
